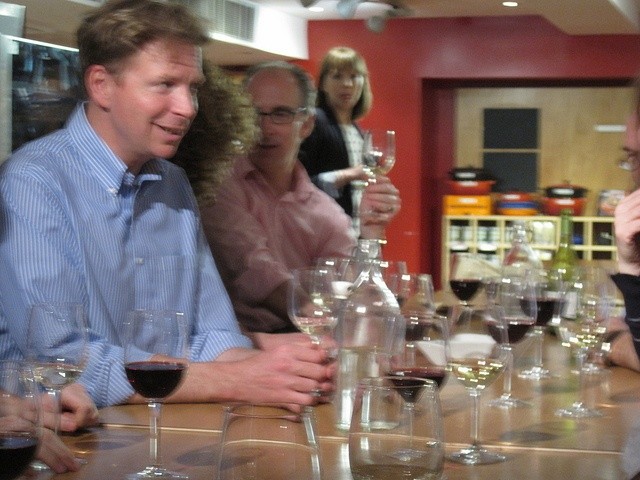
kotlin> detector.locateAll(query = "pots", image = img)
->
[496,191,543,216]
[541,182,592,216]
[443,167,497,196]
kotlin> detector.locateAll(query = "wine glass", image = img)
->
[359,127,397,217]
[286,236,451,480]
[123,309,190,479]
[388,209,608,466]
[24,301,90,470]
[0,360,44,478]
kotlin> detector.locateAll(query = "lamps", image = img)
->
[336,0,413,33]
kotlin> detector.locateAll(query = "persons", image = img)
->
[199,62,447,336]
[166,59,402,375]
[0,1,343,410]
[296,45,382,276]
[572,81,640,373]
[0,382,102,475]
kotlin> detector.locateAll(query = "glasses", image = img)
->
[615,152,640,171]
[255,107,307,124]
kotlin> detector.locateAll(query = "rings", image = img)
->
[312,382,323,398]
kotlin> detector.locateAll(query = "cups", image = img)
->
[216,402,322,480]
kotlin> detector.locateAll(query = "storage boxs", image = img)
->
[444,195,496,216]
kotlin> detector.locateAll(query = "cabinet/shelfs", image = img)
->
[439,213,618,291]
